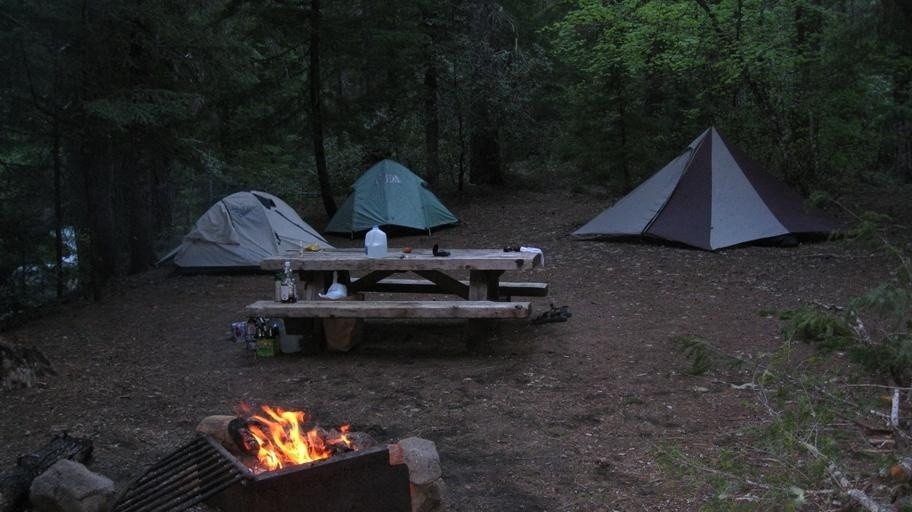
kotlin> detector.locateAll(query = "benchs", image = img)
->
[333,276,549,302]
[244,299,533,359]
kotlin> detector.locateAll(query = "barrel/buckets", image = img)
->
[254,318,280,359]
[363,224,389,258]
[280,261,298,304]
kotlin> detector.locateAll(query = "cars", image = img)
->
[6,223,80,290]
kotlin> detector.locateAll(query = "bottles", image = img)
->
[273,261,297,302]
[256,315,278,336]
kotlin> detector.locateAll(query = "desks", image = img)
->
[259,239,539,359]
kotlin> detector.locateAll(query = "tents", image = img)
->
[320,159,461,239]
[567,126,852,252]
[156,191,334,271]
